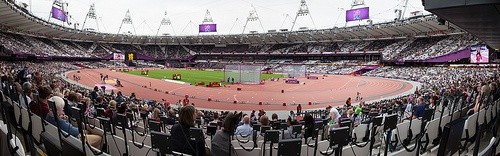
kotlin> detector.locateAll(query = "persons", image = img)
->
[0,32,500,156]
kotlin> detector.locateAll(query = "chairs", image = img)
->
[0,85,500,156]
